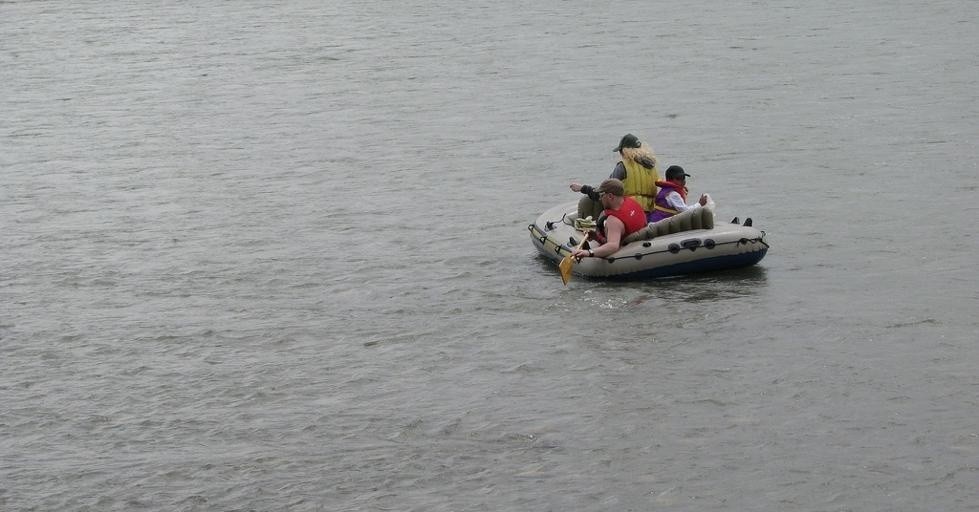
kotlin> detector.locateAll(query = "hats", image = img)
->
[666,165,690,178]
[593,178,624,194]
[612,134,641,152]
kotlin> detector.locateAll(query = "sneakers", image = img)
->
[744,218,752,226]
[731,217,738,224]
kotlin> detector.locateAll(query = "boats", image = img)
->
[526,192,774,284]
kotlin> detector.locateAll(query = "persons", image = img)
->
[570,133,708,258]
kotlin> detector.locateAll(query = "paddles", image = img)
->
[559,232,589,285]
